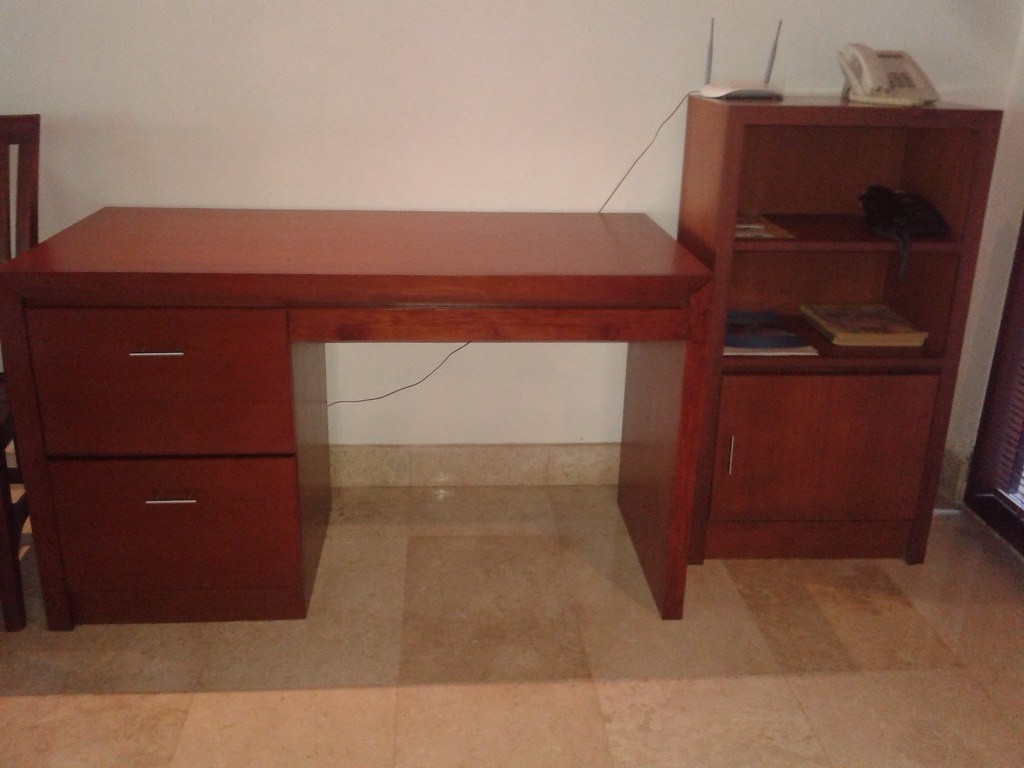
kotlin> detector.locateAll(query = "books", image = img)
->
[800,302,928,347]
[722,309,819,355]
[735,209,790,236]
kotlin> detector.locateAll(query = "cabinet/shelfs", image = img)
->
[677,91,1003,565]
[25,308,332,625]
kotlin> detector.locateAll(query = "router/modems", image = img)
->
[700,18,786,104]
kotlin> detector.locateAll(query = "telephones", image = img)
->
[834,43,940,105]
[859,183,952,238]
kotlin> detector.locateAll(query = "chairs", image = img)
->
[0,113,41,633]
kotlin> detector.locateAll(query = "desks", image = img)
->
[0,205,713,631]
